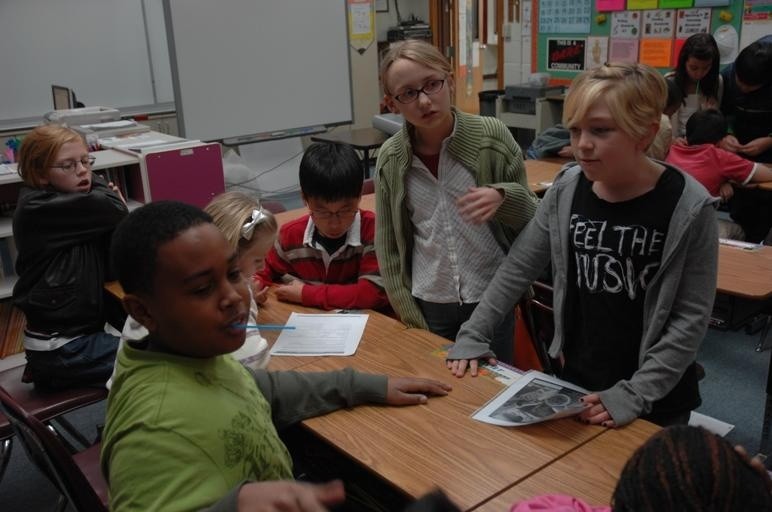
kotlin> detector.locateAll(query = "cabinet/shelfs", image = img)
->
[491,92,562,157]
[0,120,226,301]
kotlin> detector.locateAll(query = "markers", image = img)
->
[236,135,255,140]
[270,131,287,137]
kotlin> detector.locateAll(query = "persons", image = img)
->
[248,138,391,311]
[505,429,772,512]
[532,115,576,160]
[112,191,278,393]
[369,38,534,365]
[99,200,452,511]
[443,56,723,434]
[637,33,771,253]
[11,123,133,390]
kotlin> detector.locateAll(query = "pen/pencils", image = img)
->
[241,325,297,330]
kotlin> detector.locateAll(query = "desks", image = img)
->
[268,158,565,248]
[704,237,770,354]
[307,124,390,178]
[452,413,771,512]
[100,271,409,372]
[287,327,612,512]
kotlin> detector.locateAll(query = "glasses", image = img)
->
[50,155,96,173]
[394,75,447,104]
[305,200,359,217]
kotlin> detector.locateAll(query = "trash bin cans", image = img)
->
[479,89,518,144]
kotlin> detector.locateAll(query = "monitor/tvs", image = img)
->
[52,85,85,109]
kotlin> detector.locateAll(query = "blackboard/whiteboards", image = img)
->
[0,2,179,137]
[161,2,355,146]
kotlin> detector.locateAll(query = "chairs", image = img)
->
[0,346,112,470]
[0,385,114,506]
[258,197,288,213]
[357,175,381,197]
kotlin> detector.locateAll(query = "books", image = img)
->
[1,236,29,360]
[95,166,129,202]
[81,118,200,152]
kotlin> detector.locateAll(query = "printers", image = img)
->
[44,106,120,129]
